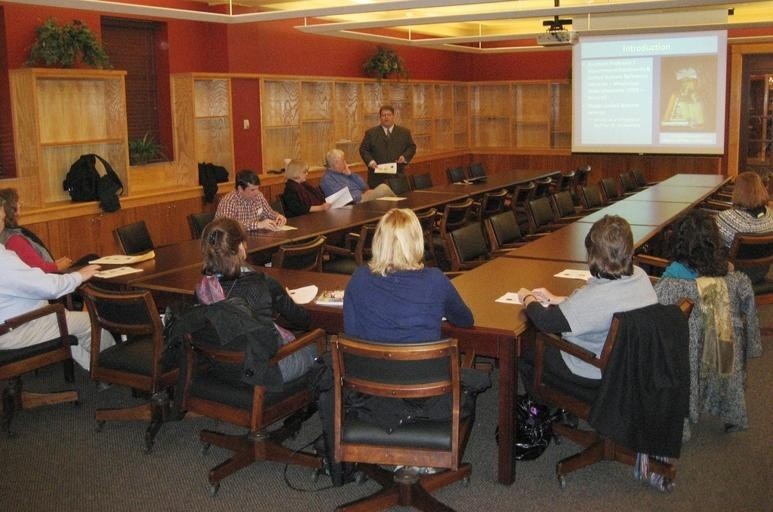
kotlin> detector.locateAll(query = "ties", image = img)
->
[384,128,392,138]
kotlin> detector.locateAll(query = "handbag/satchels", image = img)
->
[493,388,570,462]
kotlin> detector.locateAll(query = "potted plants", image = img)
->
[21,15,115,71]
[361,46,410,87]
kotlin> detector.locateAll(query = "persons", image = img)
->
[213,169,287,233]
[194,216,330,436]
[360,106,417,196]
[344,208,475,477]
[521,294,535,305]
[282,160,334,219]
[0,198,127,390]
[518,214,658,431]
[0,187,100,311]
[713,170,772,285]
[319,149,395,206]
[655,213,735,281]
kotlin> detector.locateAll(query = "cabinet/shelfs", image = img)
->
[8,68,130,213]
[170,73,237,186]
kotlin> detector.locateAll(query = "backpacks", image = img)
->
[59,150,123,203]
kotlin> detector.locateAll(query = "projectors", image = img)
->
[536,29,578,47]
